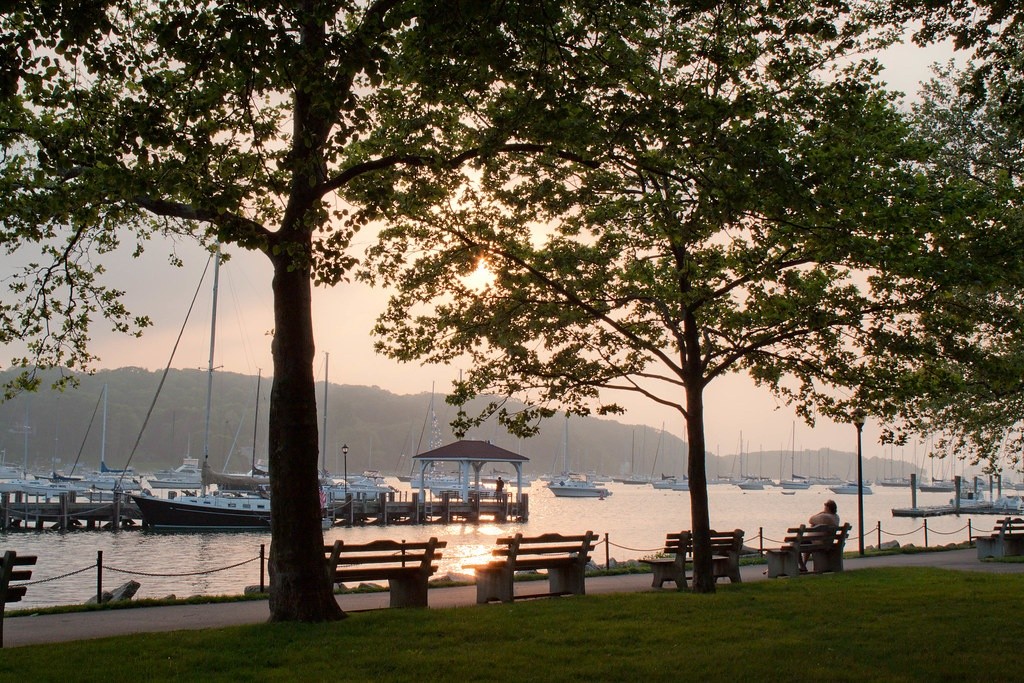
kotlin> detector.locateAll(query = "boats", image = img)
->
[508,477,532,487]
[431,485,507,498]
[542,477,613,498]
[782,491,796,495]
[480,477,510,484]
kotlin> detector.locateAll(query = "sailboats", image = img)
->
[0,239,281,530]
[539,419,611,486]
[705,420,872,490]
[310,352,487,502]
[612,421,690,491]
[829,445,873,495]
[874,433,1024,491]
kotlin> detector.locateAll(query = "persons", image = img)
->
[791,500,839,571]
[495,477,504,502]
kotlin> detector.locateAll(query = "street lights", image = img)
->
[341,443,350,493]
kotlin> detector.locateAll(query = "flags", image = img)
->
[319,480,326,509]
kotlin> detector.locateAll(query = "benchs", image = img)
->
[638,529,744,588]
[0,550,37,649]
[462,531,599,603]
[758,522,852,578]
[970,517,1024,560]
[324,537,447,608]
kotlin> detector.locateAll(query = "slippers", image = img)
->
[798,568,808,572]
